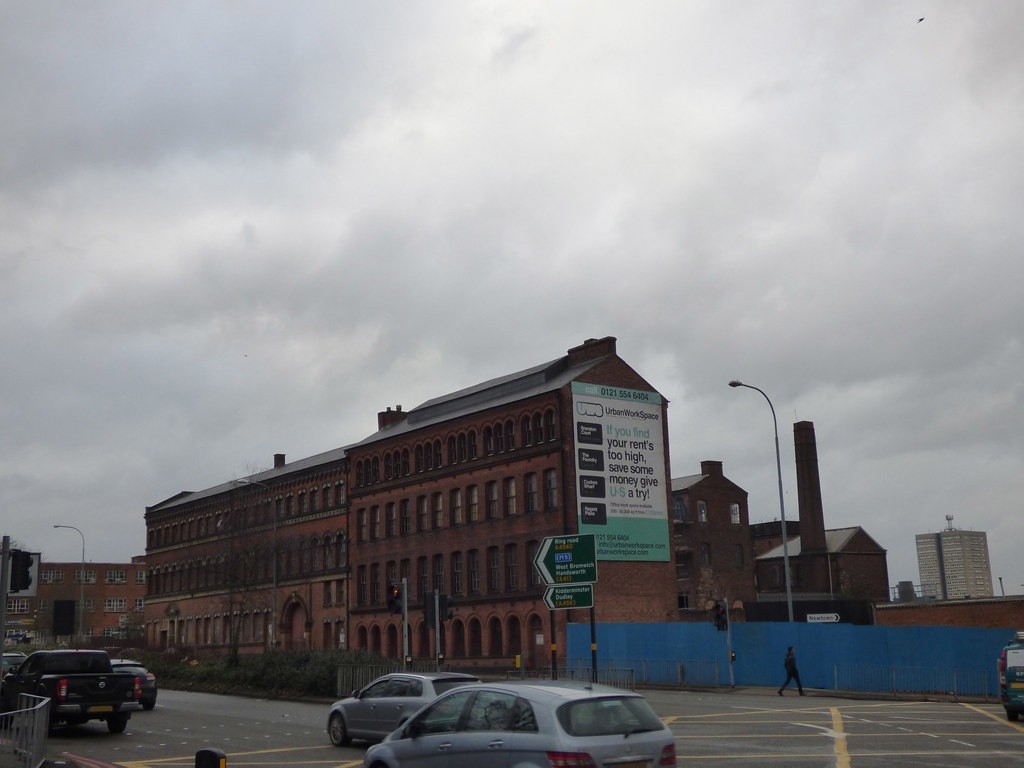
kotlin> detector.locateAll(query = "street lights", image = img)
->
[53,525,85,645]
[729,381,795,622]
[236,480,276,653]
[5,590,20,638]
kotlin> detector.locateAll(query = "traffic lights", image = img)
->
[387,581,403,598]
[10,549,34,591]
[439,592,456,622]
[713,601,728,630]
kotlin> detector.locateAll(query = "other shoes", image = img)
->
[777,691,784,697]
[799,692,806,696]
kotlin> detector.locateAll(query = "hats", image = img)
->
[787,646,795,652]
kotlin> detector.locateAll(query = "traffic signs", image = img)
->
[543,584,594,610]
[534,533,598,586]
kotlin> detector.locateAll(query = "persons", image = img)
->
[777,646,806,696]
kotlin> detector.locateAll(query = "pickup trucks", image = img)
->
[2,649,139,734]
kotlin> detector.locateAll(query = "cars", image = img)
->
[2,652,28,680]
[361,679,677,768]
[327,672,486,747]
[109,658,158,711]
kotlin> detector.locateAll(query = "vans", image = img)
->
[997,633,1024,722]
